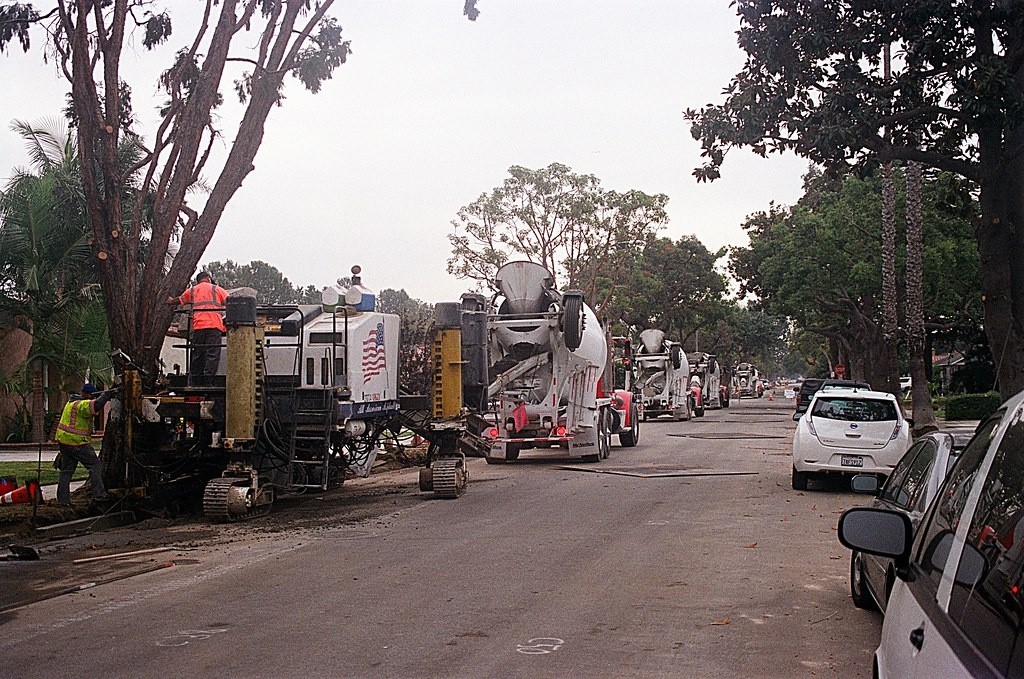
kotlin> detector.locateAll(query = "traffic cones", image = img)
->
[767,392,775,402]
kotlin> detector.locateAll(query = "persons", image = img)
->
[166,272,228,386]
[56,383,118,507]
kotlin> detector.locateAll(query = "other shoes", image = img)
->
[57,503,77,507]
[95,495,110,501]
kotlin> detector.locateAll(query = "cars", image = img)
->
[834,386,1023,679]
[792,378,827,411]
[791,389,917,492]
[820,379,873,392]
[900,376,911,395]
[848,423,983,619]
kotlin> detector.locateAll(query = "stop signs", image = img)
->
[834,364,848,376]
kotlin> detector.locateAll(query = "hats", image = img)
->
[197,272,216,284]
[81,384,98,396]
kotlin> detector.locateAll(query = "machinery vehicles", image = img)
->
[471,260,642,459]
[619,329,773,422]
[99,264,473,518]
